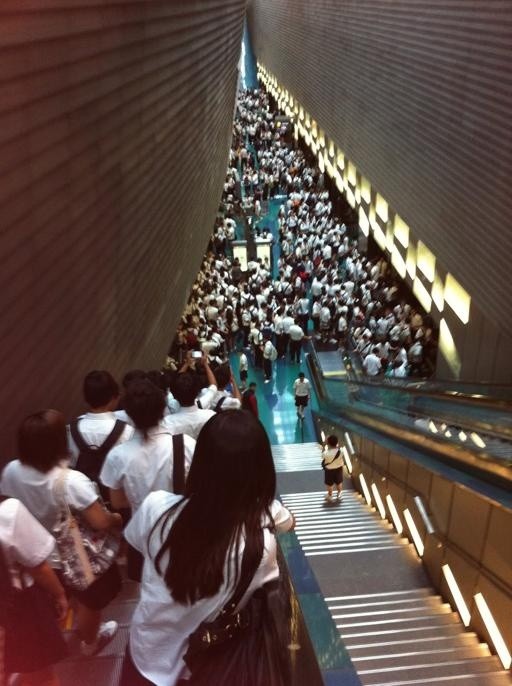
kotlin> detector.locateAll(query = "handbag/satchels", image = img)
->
[319,310,350,336]
[0,467,122,672]
[322,460,325,470]
[181,517,292,686]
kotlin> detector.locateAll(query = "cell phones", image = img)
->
[191,350,203,358]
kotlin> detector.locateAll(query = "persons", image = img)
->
[165,91,437,391]
[293,372,311,420]
[321,434,346,502]
[1,347,295,685]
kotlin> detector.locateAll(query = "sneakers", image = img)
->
[326,495,343,501]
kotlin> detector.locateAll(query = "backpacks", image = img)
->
[185,328,200,345]
[69,418,127,501]
[217,262,302,361]
